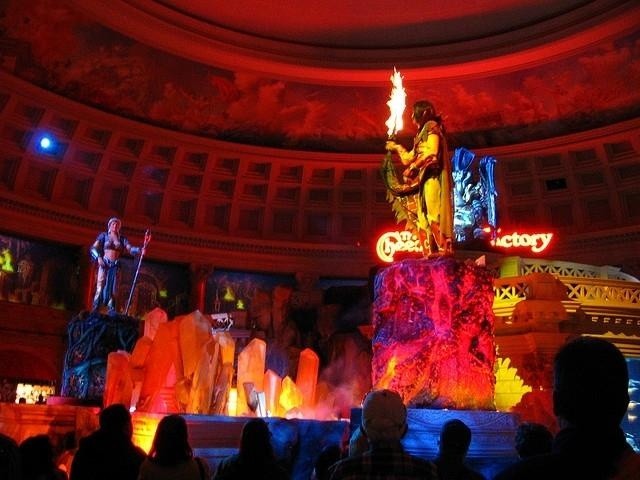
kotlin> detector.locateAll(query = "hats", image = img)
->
[361,389,408,442]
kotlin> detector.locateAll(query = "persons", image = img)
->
[1,338,640,479]
[385,100,456,261]
[90,218,146,316]
